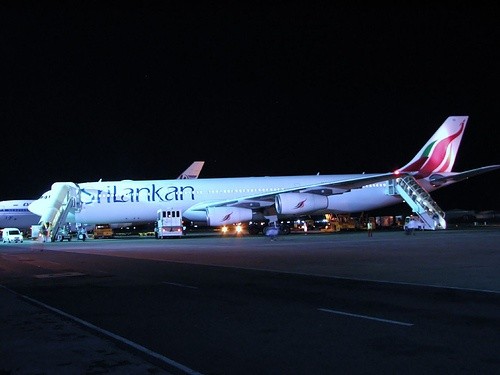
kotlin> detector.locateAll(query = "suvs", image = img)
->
[2,228,24,243]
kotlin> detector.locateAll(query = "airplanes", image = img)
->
[27,115,500,236]
[0,161,205,240]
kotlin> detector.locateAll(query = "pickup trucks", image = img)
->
[93,224,113,239]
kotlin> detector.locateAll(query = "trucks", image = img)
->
[154,209,183,239]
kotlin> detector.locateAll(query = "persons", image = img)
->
[82,228,86,242]
[154,227,158,239]
[367,220,373,237]
[42,229,47,243]
